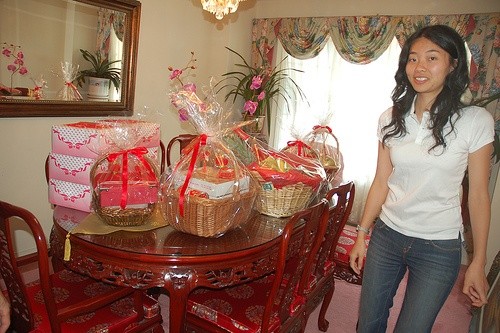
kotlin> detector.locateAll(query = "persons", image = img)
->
[348,25,494,333]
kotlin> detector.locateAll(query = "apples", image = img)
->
[321,155,335,167]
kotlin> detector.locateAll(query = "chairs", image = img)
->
[183,197,330,333]
[166,134,197,170]
[0,202,158,333]
[272,181,356,333]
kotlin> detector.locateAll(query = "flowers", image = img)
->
[2,42,28,98]
[204,45,312,137]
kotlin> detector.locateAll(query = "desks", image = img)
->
[48,190,349,333]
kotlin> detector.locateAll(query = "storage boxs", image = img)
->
[96,170,159,208]
[47,119,162,213]
[174,167,250,197]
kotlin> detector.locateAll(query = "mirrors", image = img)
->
[0,0,142,118]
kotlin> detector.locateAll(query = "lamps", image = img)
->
[201,0,247,21]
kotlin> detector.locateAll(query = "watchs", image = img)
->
[356,224,368,234]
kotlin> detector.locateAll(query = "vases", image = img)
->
[241,113,265,138]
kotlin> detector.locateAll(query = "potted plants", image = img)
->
[72,48,122,99]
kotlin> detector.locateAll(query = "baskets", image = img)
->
[81,219,293,256]
[90,148,159,227]
[279,129,341,204]
[159,140,261,237]
[246,142,327,218]
